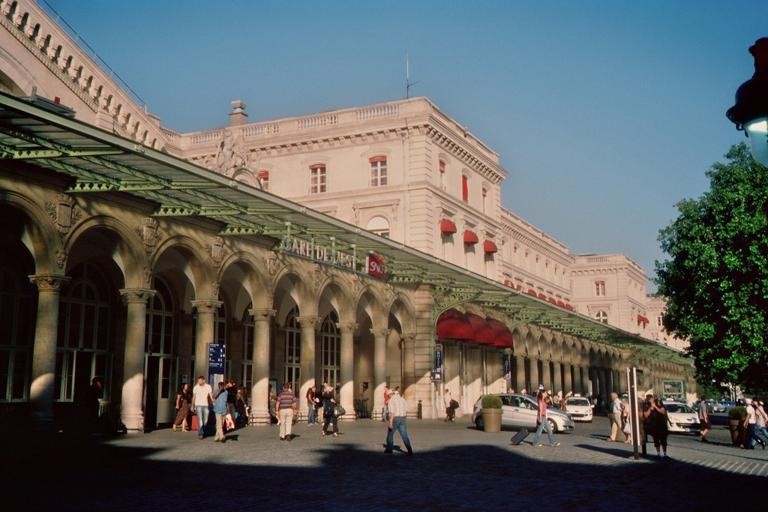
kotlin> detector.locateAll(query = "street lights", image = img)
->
[722,35,767,172]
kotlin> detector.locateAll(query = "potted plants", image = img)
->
[482,393,503,433]
[726,405,747,447]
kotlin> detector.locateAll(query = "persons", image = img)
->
[445,389,455,421]
[637,388,767,456]
[82,377,112,451]
[172,376,413,457]
[508,387,633,447]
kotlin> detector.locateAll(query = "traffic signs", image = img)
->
[208,342,225,375]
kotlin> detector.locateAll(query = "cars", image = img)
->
[561,396,595,422]
[657,398,702,432]
[471,393,575,433]
[692,400,738,413]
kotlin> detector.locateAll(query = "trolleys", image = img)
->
[443,388,459,422]
